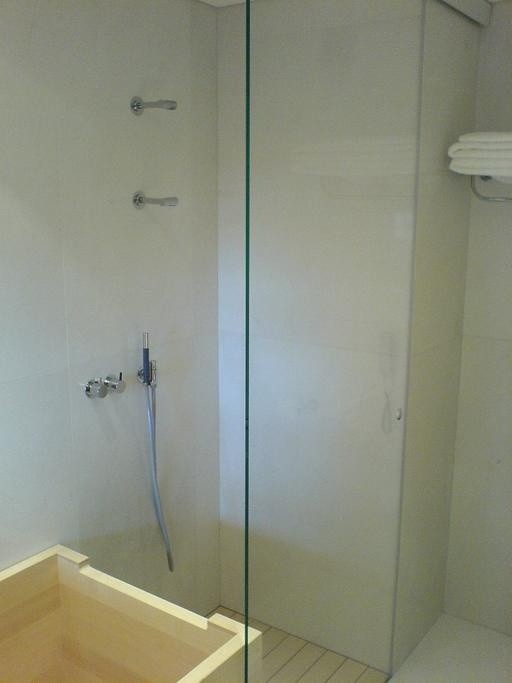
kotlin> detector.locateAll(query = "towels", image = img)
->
[446,130,511,177]
